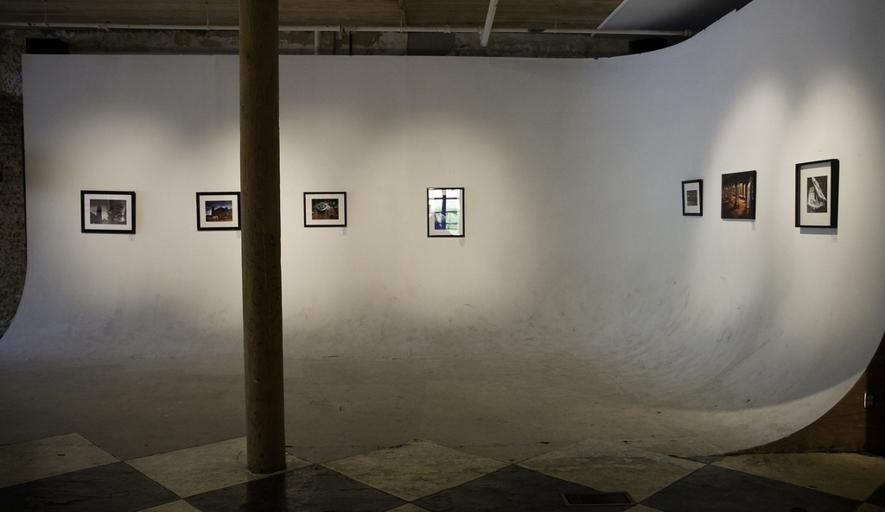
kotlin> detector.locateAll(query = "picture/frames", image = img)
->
[195,191,241,230]
[303,192,347,227]
[681,179,703,216]
[795,160,839,229]
[426,187,465,238]
[80,190,136,234]
[720,170,757,219]
